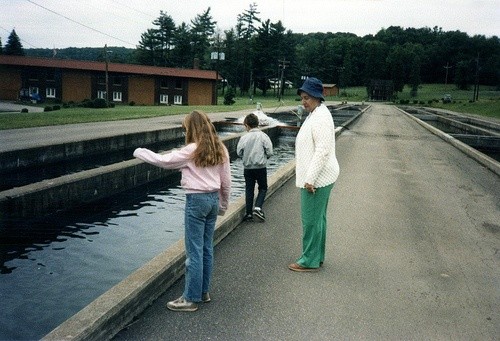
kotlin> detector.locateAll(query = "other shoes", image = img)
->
[253,210,265,221]
[244,214,252,221]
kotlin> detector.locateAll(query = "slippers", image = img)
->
[289,258,323,272]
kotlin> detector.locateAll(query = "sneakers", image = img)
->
[201,292,211,302]
[167,295,198,312]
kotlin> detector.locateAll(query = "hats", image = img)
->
[297,77,325,101]
